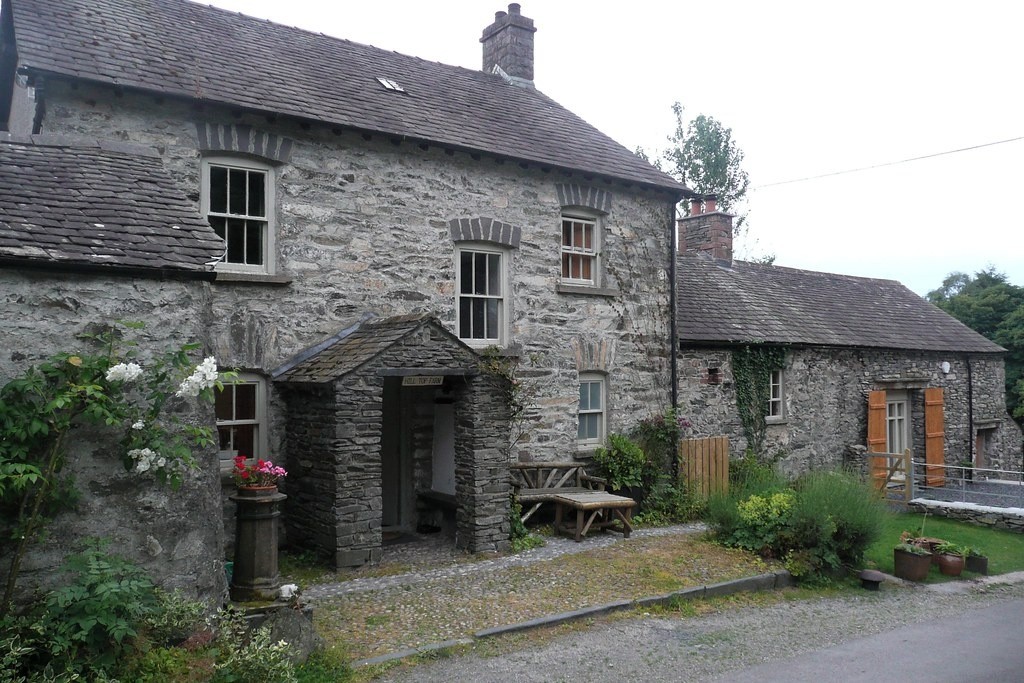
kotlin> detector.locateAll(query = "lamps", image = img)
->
[936,362,950,378]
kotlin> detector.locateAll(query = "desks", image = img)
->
[553,492,636,544]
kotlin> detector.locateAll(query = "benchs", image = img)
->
[508,461,606,535]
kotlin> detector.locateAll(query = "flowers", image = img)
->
[280,580,301,600]
[227,456,285,487]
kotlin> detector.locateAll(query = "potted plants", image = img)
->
[894,544,932,583]
[964,547,988,574]
[937,541,964,577]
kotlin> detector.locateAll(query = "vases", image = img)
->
[238,484,278,497]
